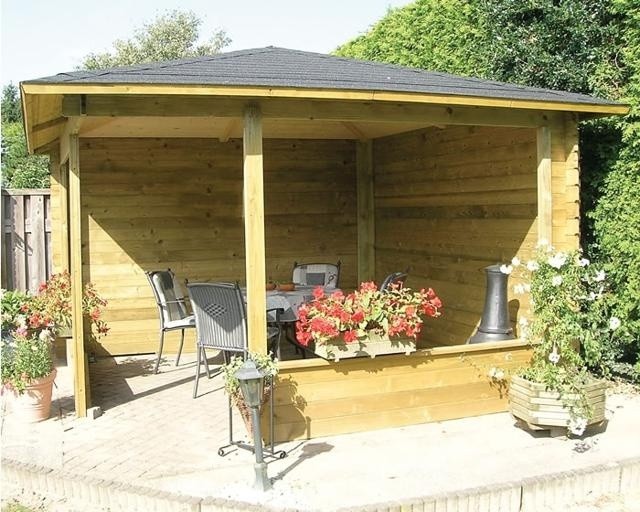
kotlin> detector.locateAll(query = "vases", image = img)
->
[507,369,609,430]
[9,368,57,420]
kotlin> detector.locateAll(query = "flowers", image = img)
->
[222,347,282,403]
[0,269,112,395]
[487,235,630,436]
[292,275,444,365]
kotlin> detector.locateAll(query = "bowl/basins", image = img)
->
[266,283,294,291]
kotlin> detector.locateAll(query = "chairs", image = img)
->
[146,258,409,403]
